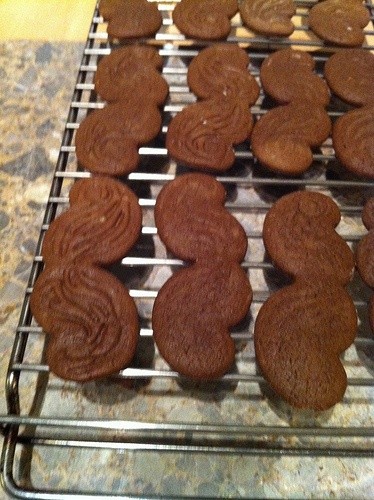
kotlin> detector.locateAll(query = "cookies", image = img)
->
[29,0,374,409]
